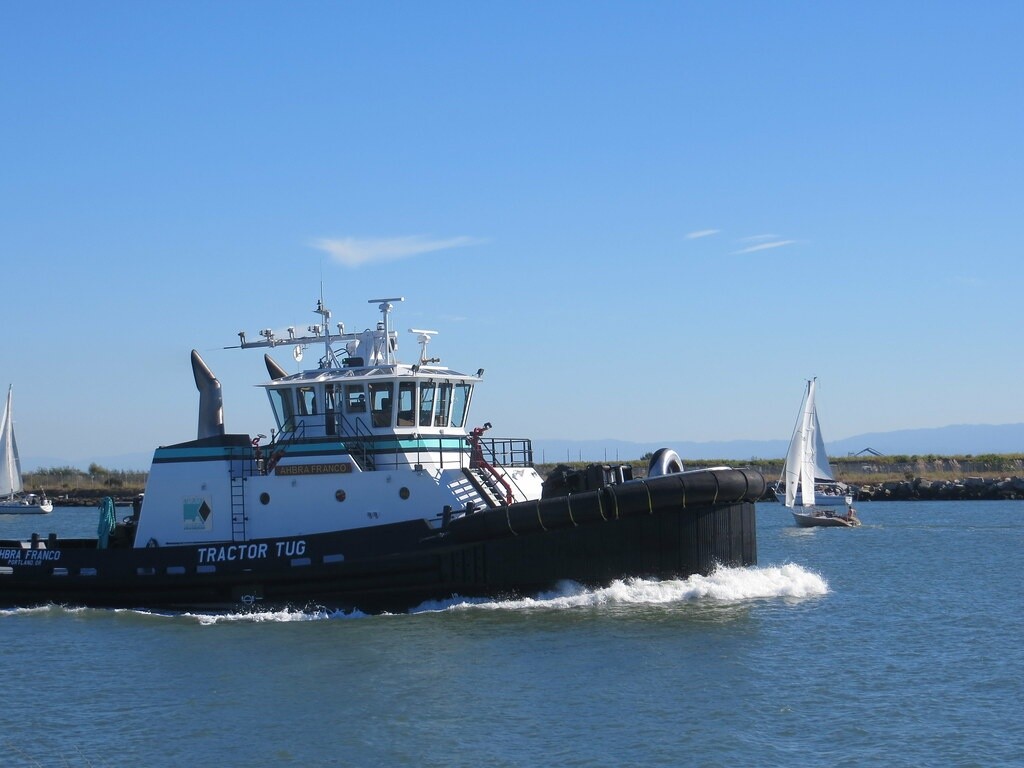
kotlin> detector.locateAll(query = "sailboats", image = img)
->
[0,382,54,515]
[771,372,865,529]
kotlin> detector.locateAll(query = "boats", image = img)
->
[772,477,855,505]
[0,280,777,624]
